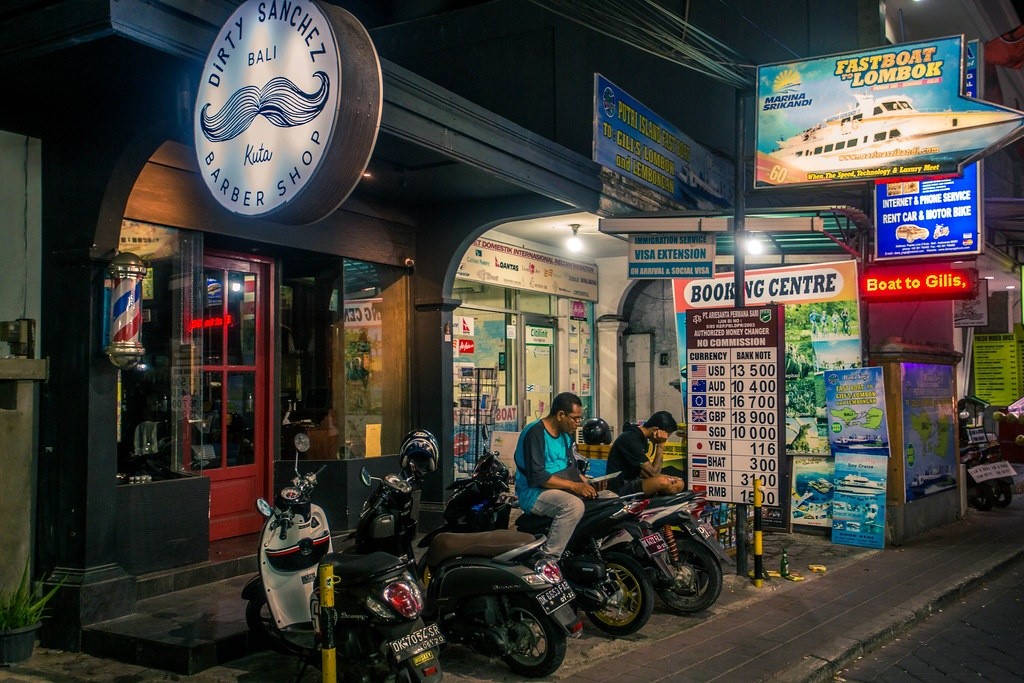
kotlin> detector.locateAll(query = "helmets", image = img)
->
[583,418,611,445]
[400,429,439,475]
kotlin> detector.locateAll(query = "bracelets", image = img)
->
[579,484,585,495]
[657,442,664,448]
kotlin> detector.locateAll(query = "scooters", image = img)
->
[960,441,1015,511]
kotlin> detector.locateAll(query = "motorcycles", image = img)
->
[342,429,590,679]
[240,431,447,682]
[447,436,734,635]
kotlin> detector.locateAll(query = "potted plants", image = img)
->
[0,554,70,663]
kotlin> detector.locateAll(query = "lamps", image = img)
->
[103,252,151,367]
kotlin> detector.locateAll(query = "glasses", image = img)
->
[566,412,584,421]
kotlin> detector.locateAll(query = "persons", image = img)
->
[514,392,620,584]
[605,410,685,496]
